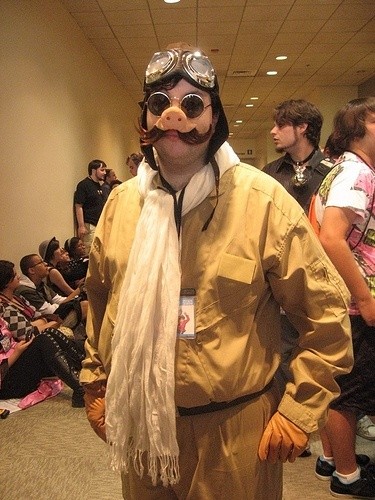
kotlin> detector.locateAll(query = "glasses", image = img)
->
[26,260,46,269]
[144,91,212,118]
[139,47,216,90]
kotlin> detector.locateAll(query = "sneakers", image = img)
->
[356,415,375,439]
[313,451,375,497]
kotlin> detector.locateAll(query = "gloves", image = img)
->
[83,384,107,441]
[257,412,307,463]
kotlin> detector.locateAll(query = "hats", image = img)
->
[38,236,55,258]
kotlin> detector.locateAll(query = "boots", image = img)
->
[48,350,85,408]
